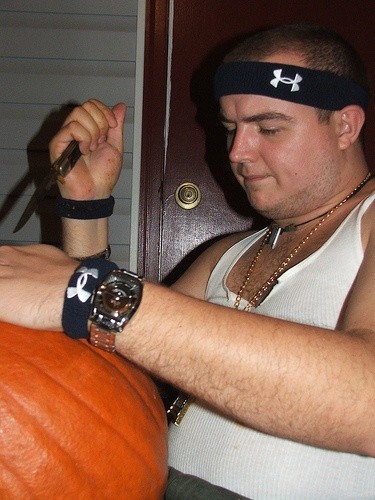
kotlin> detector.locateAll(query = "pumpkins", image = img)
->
[0,321,170,500]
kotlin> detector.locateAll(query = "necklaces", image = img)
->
[165,169,373,428]
[265,170,375,249]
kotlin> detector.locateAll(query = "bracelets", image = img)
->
[61,258,119,338]
[57,196,116,219]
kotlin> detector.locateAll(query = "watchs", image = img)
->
[89,267,144,353]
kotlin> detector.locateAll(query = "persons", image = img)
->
[1,22,375,496]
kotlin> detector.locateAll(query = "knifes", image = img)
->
[13,139,82,234]
